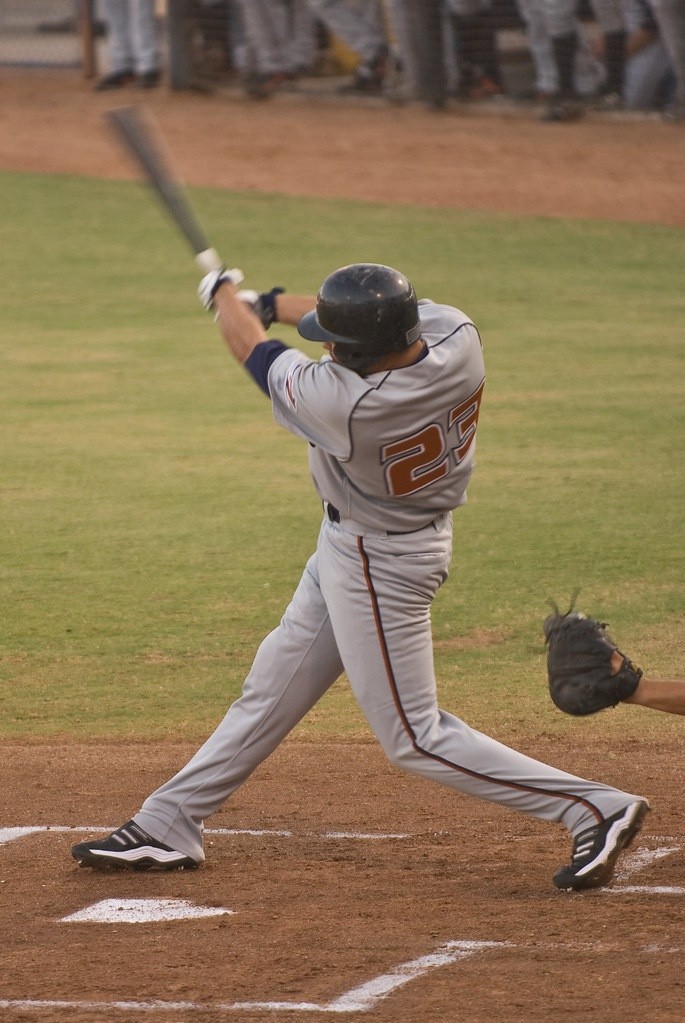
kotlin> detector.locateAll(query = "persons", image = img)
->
[89,1,684,126]
[72,263,650,891]
[544,584,684,718]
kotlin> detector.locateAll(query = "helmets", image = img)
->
[298,262,423,366]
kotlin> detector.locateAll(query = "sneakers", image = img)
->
[70,819,206,868]
[552,800,648,889]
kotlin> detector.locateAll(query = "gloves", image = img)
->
[197,267,285,331]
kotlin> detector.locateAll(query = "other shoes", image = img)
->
[98,69,161,90]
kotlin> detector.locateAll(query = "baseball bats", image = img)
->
[95,101,220,272]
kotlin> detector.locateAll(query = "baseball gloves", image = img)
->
[543,584,646,718]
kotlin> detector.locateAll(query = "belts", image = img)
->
[321,500,434,535]
